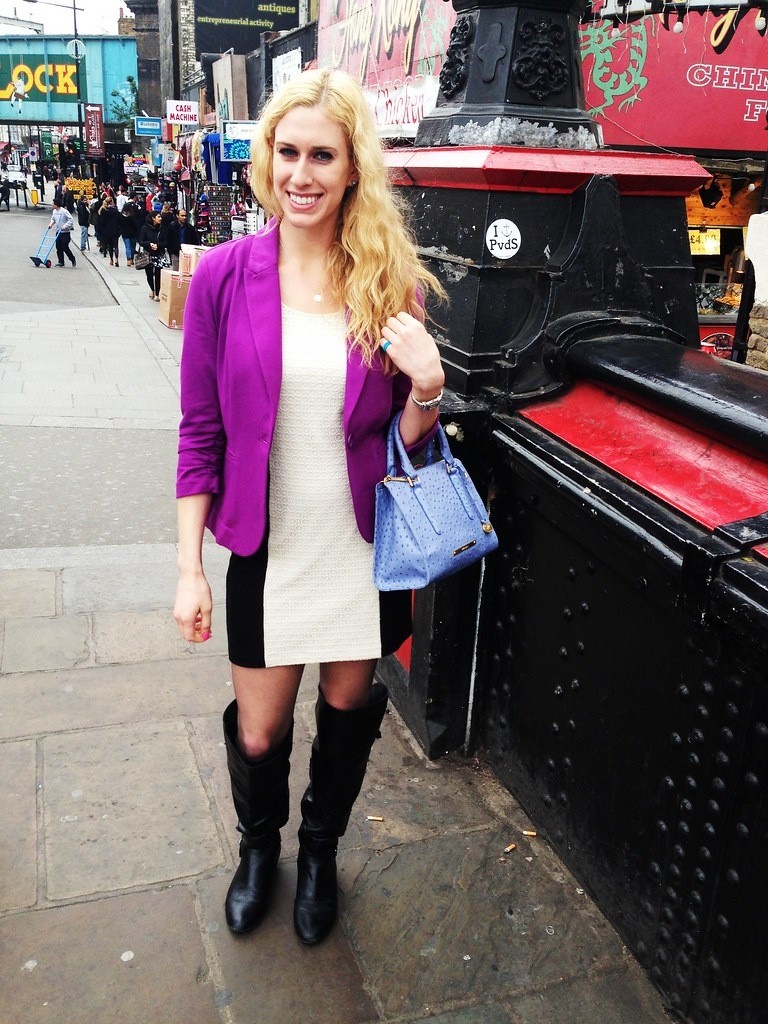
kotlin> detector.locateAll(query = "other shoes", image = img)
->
[131,259,133,265]
[104,253,106,257]
[149,290,154,298]
[115,263,119,267]
[56,262,64,267]
[110,260,113,264]
[154,295,160,301]
[82,249,91,252]
[72,258,76,266]
[127,259,131,266]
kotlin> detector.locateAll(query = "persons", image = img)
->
[48,199,76,267]
[1,161,7,171]
[23,165,28,178]
[174,70,445,946]
[37,165,41,174]
[44,165,198,300]
[0,180,10,211]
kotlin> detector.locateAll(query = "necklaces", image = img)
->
[280,240,333,302]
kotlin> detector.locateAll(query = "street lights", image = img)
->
[23,0,84,180]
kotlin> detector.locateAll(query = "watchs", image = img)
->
[411,389,444,411]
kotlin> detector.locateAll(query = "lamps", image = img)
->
[698,184,723,209]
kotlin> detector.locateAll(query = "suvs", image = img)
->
[1,165,20,184]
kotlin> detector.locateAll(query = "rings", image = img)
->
[382,340,391,351]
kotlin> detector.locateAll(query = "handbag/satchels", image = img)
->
[134,240,152,270]
[372,408,501,592]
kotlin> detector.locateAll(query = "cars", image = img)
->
[4,172,26,188]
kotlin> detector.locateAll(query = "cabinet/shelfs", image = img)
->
[203,184,235,244]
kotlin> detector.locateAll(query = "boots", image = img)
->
[293,683,390,946]
[223,699,294,934]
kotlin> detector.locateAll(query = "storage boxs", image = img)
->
[158,269,191,330]
[191,246,213,275]
[178,244,193,275]
